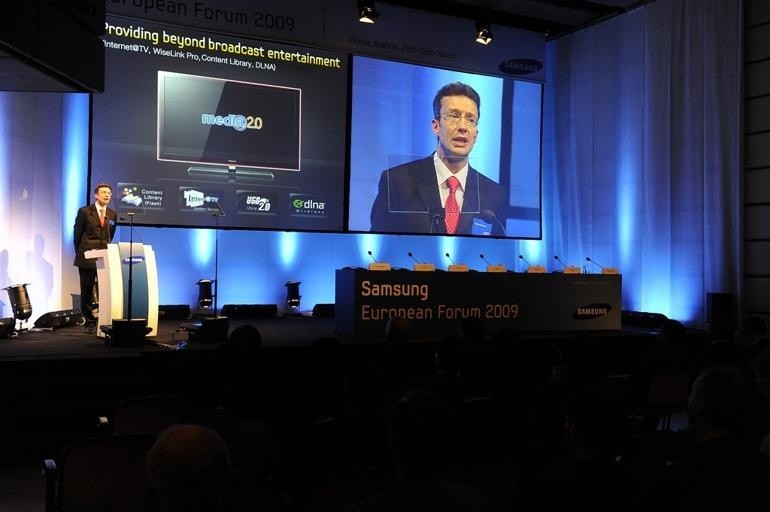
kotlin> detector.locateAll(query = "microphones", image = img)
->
[446,253,455,265]
[480,254,490,266]
[519,255,532,267]
[408,252,420,264]
[586,257,607,269]
[213,213,219,217]
[368,251,376,263]
[480,209,507,236]
[120,216,143,243]
[554,256,566,267]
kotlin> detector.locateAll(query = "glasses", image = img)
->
[437,113,478,129]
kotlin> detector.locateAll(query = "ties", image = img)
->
[444,177,464,234]
[100,209,105,227]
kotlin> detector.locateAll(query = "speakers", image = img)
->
[0,318,16,340]
[312,304,335,319]
[706,293,739,332]
[221,304,277,319]
[34,309,81,330]
[159,305,190,320]
[0,0,106,95]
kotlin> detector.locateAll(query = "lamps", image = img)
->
[0,282,37,335]
[358,0,385,24]
[283,280,304,316]
[475,15,494,46]
[194,277,219,313]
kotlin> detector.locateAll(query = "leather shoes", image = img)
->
[83,313,97,322]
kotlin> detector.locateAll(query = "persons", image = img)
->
[68,182,117,333]
[366,81,512,239]
[133,359,770,510]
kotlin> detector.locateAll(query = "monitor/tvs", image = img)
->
[157,70,301,179]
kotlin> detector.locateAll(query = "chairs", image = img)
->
[38,345,770,512]
